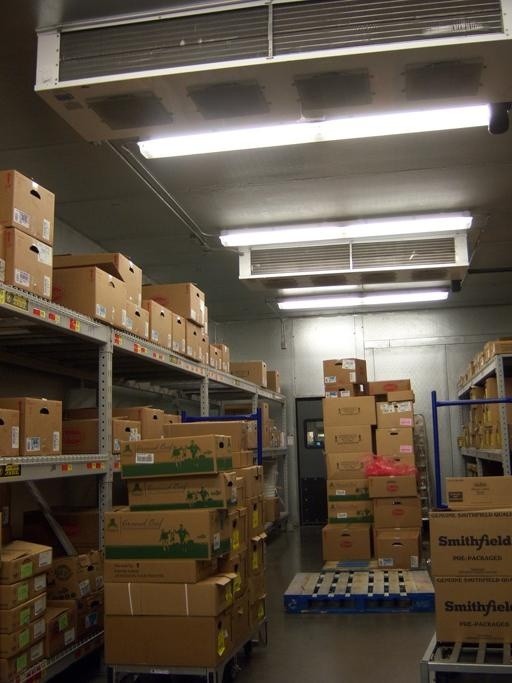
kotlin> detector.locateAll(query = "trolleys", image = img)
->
[102,404,268,683]
[421,385,511,681]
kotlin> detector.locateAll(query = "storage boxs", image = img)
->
[425,338,512,645]
[321,356,427,571]
[1,392,285,658]
[2,168,289,400]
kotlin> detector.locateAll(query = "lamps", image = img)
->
[217,210,472,250]
[137,93,512,161]
[277,286,450,312]
